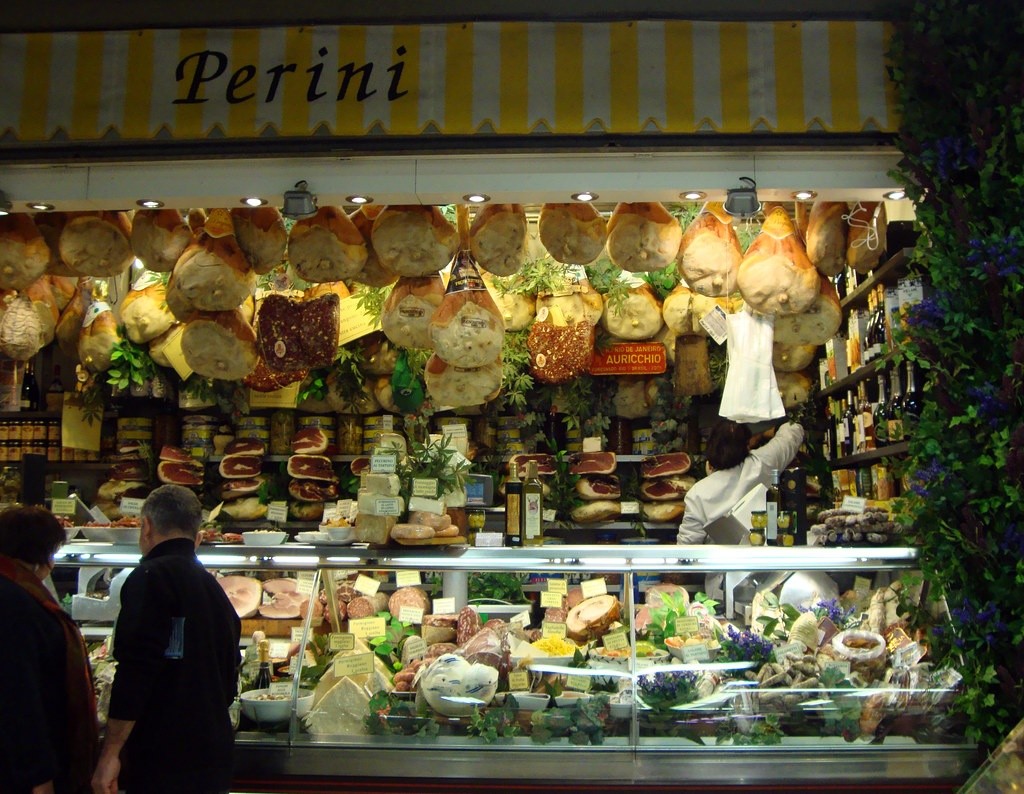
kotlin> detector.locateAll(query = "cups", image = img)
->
[466,509,485,546]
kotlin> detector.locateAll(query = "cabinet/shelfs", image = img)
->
[819,248,928,499]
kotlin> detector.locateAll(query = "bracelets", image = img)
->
[761,433,764,441]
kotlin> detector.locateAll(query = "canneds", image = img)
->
[0,419,116,462]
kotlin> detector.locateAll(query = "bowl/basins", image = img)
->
[495,692,550,710]
[507,653,580,669]
[417,678,499,717]
[732,712,782,735]
[241,530,289,546]
[240,688,315,724]
[612,704,636,718]
[588,648,670,664]
[62,527,143,545]
[318,524,354,540]
[297,531,329,539]
[295,535,359,545]
[554,690,596,709]
[664,640,723,661]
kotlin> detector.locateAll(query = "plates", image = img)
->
[203,540,232,544]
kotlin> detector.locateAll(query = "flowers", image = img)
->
[881,1,1024,748]
[307,594,872,748]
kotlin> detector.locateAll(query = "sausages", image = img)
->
[392,658,434,691]
[745,652,821,706]
[810,507,897,543]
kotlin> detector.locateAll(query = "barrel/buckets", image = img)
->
[621,537,659,583]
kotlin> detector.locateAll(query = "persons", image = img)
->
[675,418,805,600]
[91,484,242,794]
[0,503,101,794]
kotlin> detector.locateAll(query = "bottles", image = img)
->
[254,640,273,689]
[594,534,620,584]
[504,461,543,547]
[20,359,64,411]
[824,282,922,460]
[750,469,794,547]
[603,415,632,454]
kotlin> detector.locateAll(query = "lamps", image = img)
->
[723,189,762,216]
[278,181,318,219]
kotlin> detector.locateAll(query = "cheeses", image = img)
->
[354,432,457,547]
[305,639,398,737]
